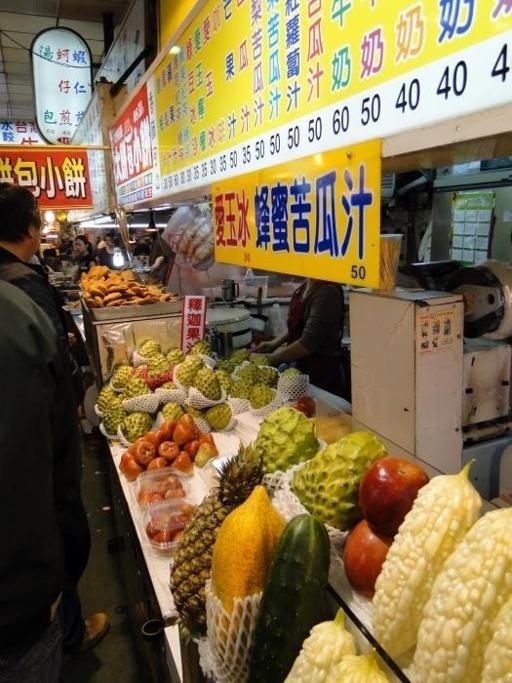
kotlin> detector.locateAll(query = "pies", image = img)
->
[80,265,176,308]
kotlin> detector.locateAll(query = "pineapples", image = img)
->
[168,438,267,638]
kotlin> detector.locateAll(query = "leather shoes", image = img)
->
[63,612,111,663]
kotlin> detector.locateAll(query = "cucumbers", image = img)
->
[249,513,332,683]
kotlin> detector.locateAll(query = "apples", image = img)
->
[345,453,430,600]
[293,396,316,418]
[119,411,218,556]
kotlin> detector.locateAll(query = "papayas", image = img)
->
[209,482,290,683]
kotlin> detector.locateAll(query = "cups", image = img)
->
[371,234,404,291]
[220,279,239,301]
[233,274,269,303]
[205,326,233,359]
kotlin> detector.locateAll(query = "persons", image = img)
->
[34,219,174,301]
[250,278,345,398]
[1,182,110,663]
[1,274,70,683]
